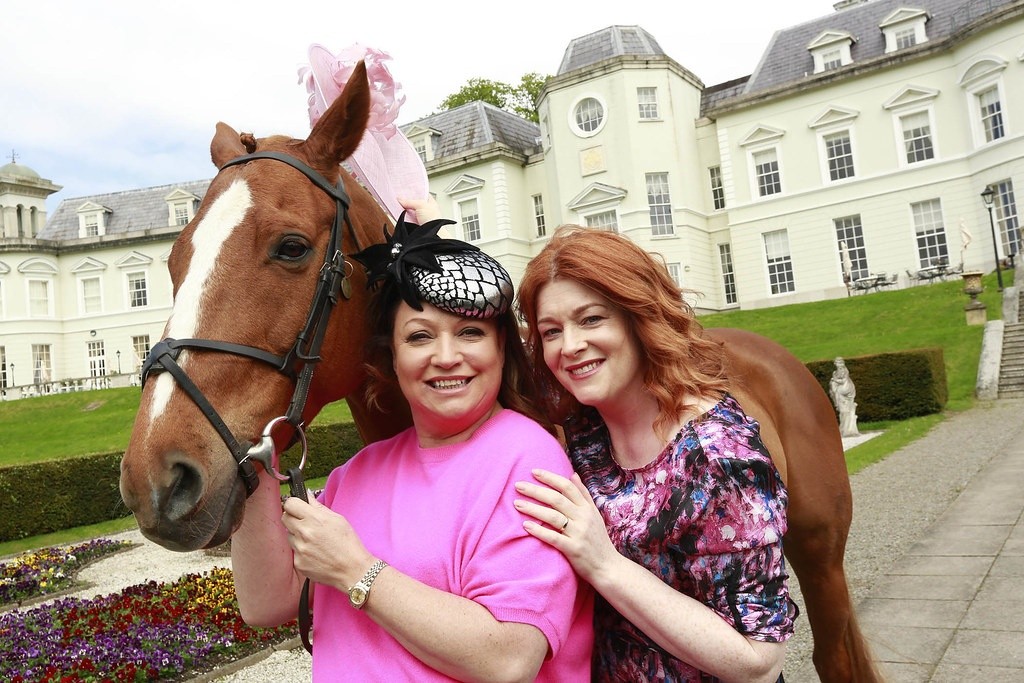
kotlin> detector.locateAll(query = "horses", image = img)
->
[117,60,888,683]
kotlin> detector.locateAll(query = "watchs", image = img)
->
[348,560,388,611]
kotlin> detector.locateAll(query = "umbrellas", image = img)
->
[840,241,853,282]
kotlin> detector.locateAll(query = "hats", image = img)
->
[299,45,429,225]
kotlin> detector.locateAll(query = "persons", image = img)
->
[231,210,594,683]
[512,225,799,683]
[829,356,859,437]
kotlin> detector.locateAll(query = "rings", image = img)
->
[559,519,569,534]
